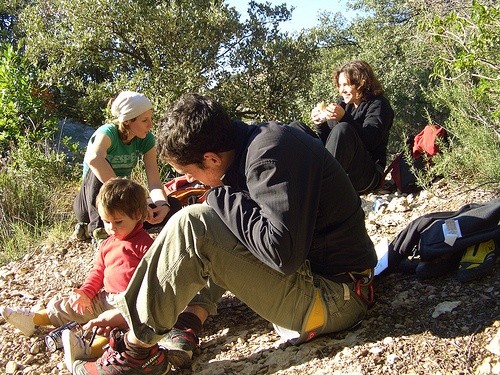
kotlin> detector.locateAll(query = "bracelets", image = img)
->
[73,94,378,375]
[160,202,170,206]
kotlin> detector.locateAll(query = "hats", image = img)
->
[111,91,152,124]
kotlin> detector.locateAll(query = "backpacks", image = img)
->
[387,201,500,281]
[163,176,211,207]
[380,124,448,194]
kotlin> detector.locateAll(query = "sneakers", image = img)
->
[74,331,169,374]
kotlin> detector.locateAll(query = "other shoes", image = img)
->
[75,222,92,240]
[92,227,109,241]
[62,329,88,374]
[0,305,38,337]
[156,329,200,364]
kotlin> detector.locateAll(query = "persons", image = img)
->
[74,89,171,242]
[2,178,154,374]
[310,61,394,193]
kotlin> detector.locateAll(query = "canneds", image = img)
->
[45,320,84,352]
